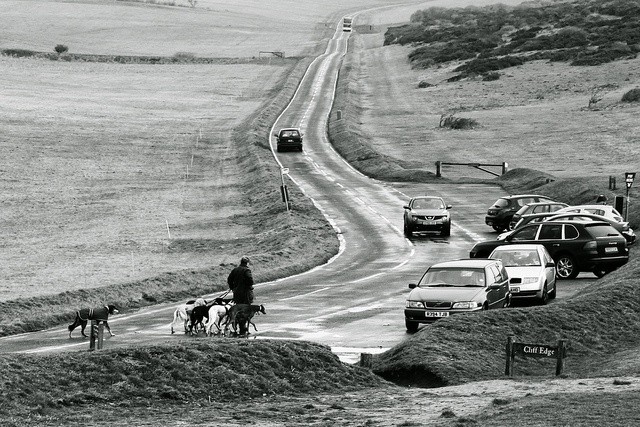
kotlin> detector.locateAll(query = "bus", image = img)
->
[344,17,352,23]
[343,23,351,32]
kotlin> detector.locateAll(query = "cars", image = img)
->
[488,244,557,305]
[542,213,636,246]
[403,195,452,237]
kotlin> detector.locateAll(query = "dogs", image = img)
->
[166,297,266,337]
[68,305,121,341]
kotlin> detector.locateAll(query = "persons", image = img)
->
[228,257,254,337]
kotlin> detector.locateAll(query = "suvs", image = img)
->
[274,127,304,152]
[404,258,513,330]
[470,220,630,280]
[485,194,560,232]
[554,205,623,223]
[511,202,570,229]
[514,213,585,230]
